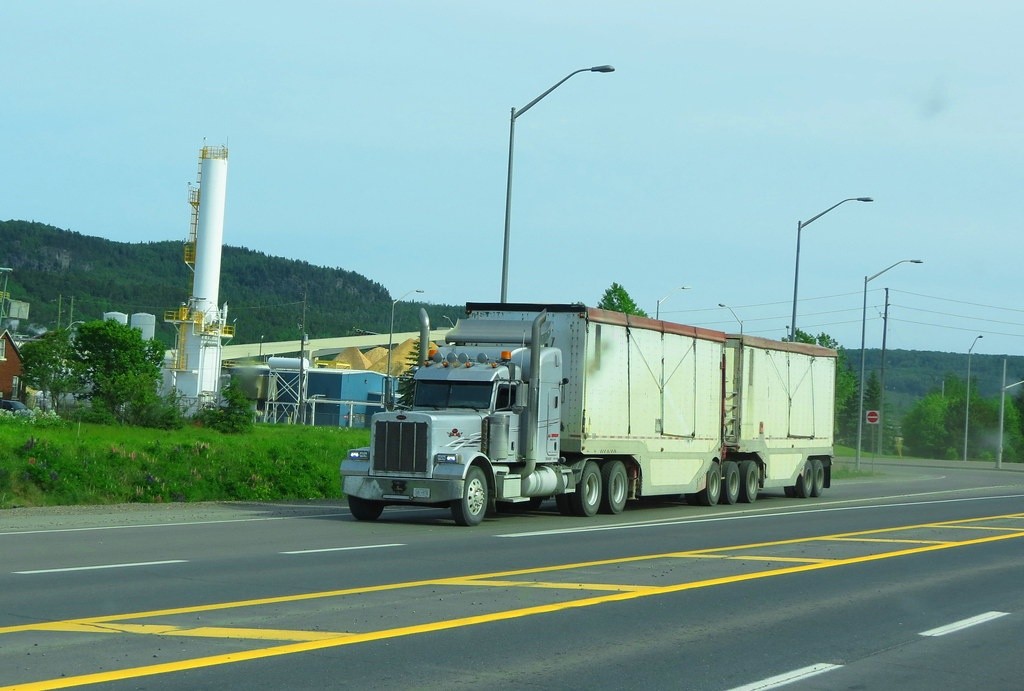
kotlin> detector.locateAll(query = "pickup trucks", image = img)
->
[0,400,29,414]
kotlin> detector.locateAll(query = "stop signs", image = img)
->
[868,412,879,422]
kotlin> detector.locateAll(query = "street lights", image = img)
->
[719,303,743,335]
[656,286,692,320]
[791,196,873,340]
[856,258,924,470]
[388,288,426,379]
[961,335,985,461]
[501,64,615,304]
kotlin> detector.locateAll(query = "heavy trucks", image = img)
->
[341,302,837,527]
